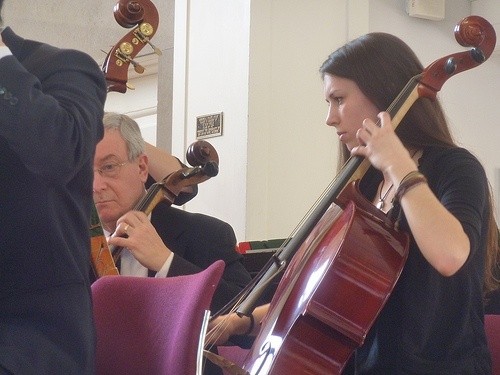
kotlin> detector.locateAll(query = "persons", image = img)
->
[205,32,500,375]
[0,26,204,375]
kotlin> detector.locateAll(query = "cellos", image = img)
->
[200,14,498,374]
[90,0,161,278]
[105,140,221,267]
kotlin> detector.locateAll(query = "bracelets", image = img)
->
[243,314,254,337]
[391,171,427,208]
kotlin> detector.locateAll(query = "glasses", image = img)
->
[92,151,141,175]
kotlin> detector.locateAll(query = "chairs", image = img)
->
[89,259,226,375]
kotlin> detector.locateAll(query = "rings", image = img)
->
[124,225,129,233]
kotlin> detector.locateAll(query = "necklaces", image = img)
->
[376,146,421,210]
